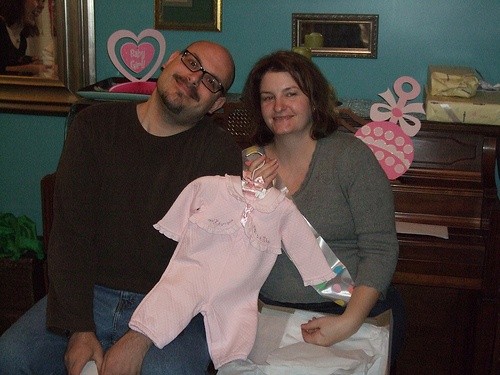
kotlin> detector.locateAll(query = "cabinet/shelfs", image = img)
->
[63,102,500,374]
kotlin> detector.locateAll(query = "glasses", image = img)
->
[181,48,225,96]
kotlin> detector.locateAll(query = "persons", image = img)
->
[216,51,399,375]
[0,0,51,75]
[0,41,243,375]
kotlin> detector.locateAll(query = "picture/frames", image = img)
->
[154,0,222,31]
[0,0,97,117]
[292,13,379,59]
